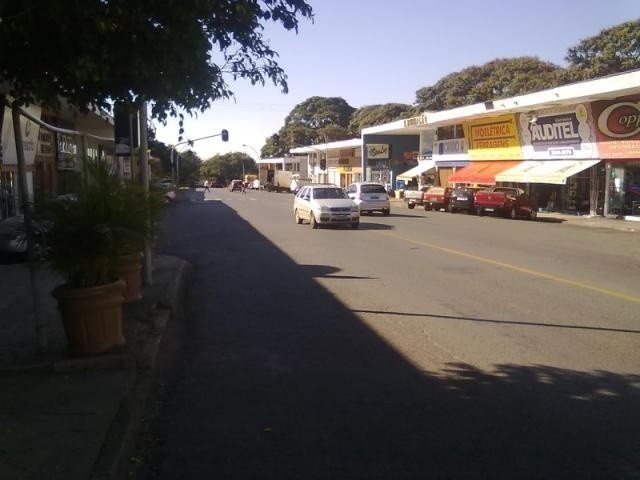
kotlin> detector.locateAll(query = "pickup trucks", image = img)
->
[476,187,537,219]
[404,186,432,209]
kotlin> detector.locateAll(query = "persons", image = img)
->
[204,180,210,192]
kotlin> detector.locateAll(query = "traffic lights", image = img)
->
[273,134,280,146]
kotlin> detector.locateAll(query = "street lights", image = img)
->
[242,143,261,163]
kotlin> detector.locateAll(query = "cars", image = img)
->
[230,180,243,191]
[424,188,449,212]
[294,184,360,229]
[450,186,485,214]
[347,182,391,216]
[0,193,79,262]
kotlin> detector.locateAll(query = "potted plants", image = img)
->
[19,155,165,356]
